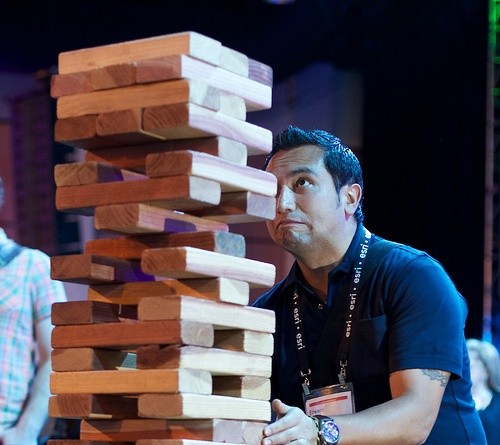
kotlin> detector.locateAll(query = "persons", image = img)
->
[465,338,500,445]
[0,225,68,444]
[236,126,487,445]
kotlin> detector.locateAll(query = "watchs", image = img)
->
[309,414,340,445]
